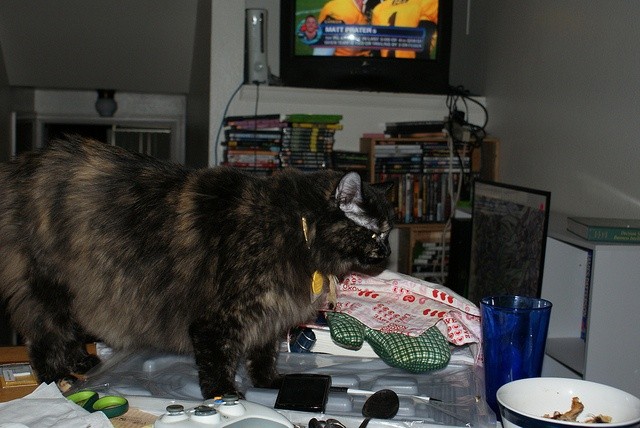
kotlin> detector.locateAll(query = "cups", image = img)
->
[479,294,554,416]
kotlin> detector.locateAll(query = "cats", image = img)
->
[0,133,396,403]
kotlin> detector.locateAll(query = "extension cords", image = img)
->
[455,125,472,143]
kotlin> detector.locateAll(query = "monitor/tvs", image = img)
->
[279,0,454,96]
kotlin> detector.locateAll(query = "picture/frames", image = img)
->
[468,178,550,314]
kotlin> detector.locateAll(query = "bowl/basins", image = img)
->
[496,375,640,427]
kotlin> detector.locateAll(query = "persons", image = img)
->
[371,0,437,58]
[298,15,321,44]
[312,0,370,55]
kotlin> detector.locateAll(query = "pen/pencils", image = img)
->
[329,385,442,403]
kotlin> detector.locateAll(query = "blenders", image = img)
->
[248,9,269,84]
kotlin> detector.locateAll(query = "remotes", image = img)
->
[383,120,444,136]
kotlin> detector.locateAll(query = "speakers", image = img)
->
[245,8,269,86]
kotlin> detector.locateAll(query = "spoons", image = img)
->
[356,388,401,428]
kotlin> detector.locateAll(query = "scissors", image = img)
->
[65,390,128,418]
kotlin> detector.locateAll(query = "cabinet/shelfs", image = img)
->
[541,209,640,393]
[358,134,499,291]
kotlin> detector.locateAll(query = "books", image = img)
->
[376,141,473,222]
[220,113,345,176]
[416,241,449,284]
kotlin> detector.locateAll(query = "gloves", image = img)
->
[324,310,451,373]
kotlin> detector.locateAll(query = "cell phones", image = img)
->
[274,373,332,413]
